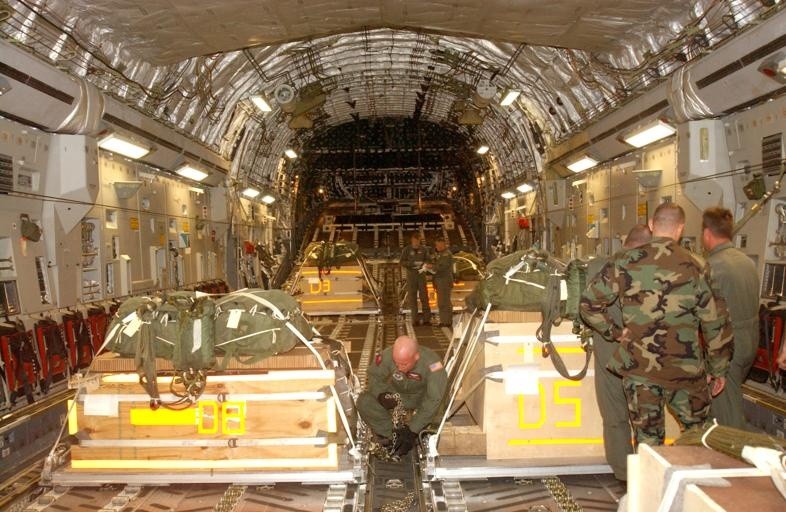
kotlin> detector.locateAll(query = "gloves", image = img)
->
[378,391,401,408]
[393,428,419,456]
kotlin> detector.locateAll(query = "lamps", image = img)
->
[89,82,300,209]
[474,86,680,202]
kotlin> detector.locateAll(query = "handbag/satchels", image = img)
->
[103,285,311,370]
[307,240,358,262]
[453,251,585,319]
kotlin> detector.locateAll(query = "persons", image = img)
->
[699,206,761,432]
[400,234,430,327]
[354,336,449,462]
[583,222,652,485]
[425,236,456,327]
[577,201,734,448]
